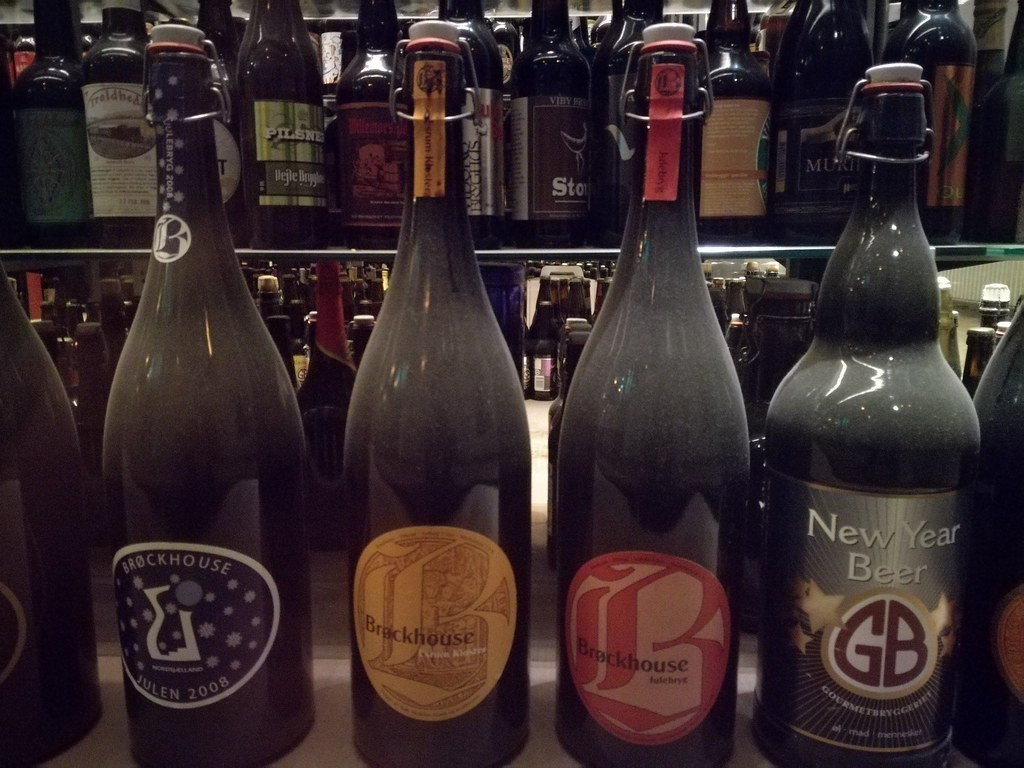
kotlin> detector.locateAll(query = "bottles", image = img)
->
[0,0,1024,768]
